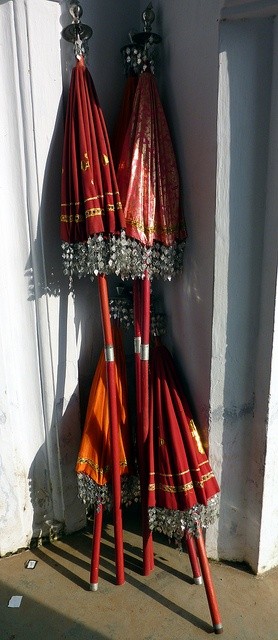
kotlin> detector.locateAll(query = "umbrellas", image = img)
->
[148,289,224,635]
[114,0,189,578]
[75,286,134,592]
[58,0,129,587]
